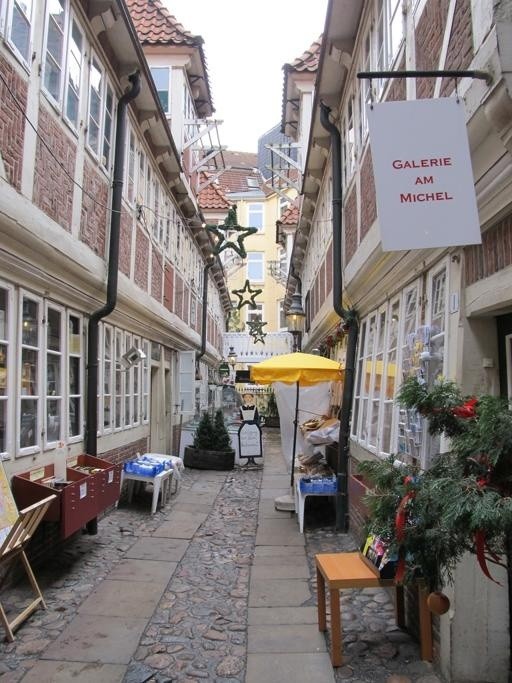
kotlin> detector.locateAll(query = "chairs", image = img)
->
[315,551,434,667]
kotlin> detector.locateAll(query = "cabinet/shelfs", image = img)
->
[0,458,57,643]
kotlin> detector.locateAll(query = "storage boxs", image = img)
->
[12,452,123,540]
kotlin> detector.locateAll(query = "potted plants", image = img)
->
[265,392,280,428]
[184,409,235,471]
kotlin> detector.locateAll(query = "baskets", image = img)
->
[124,456,172,477]
[300,475,338,493]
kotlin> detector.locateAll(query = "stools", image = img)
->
[293,473,337,534]
[114,452,183,514]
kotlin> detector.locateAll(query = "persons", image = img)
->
[239,393,262,458]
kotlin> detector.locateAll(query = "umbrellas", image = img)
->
[250,350,345,496]
